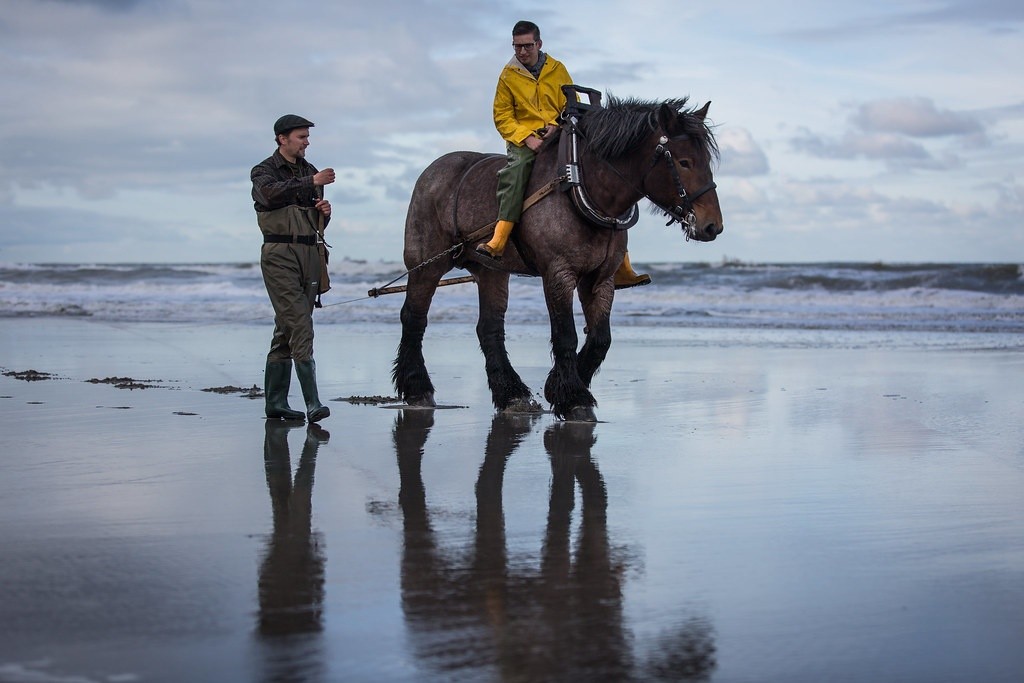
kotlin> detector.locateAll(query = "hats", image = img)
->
[274,114,314,135]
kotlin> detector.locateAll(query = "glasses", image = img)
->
[512,40,538,51]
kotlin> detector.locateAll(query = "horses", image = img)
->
[390,99,726,424]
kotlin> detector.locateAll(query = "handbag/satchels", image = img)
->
[314,241,332,308]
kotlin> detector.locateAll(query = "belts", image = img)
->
[264,235,315,245]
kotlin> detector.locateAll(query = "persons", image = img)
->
[251,113,336,425]
[475,19,651,290]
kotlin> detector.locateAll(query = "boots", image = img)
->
[265,361,305,420]
[614,251,652,289]
[294,360,330,424]
[475,220,515,264]
